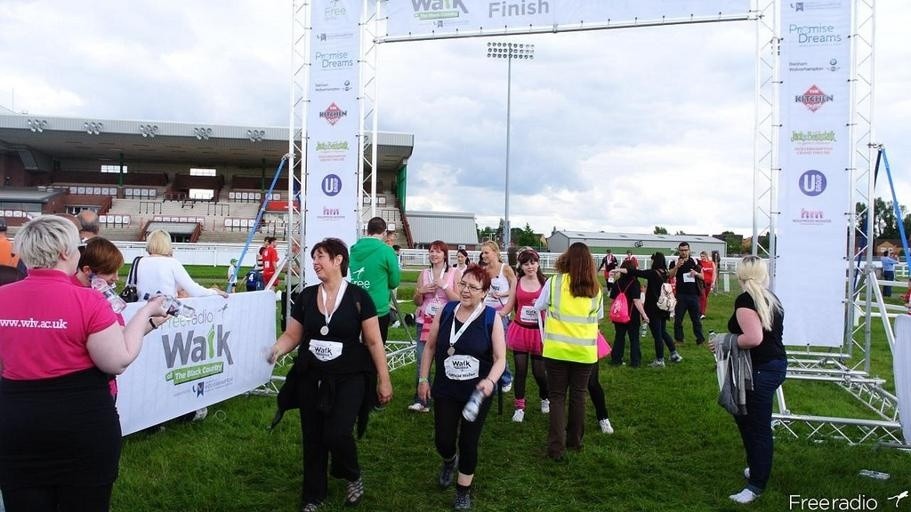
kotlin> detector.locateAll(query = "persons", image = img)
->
[531,240,605,462]
[0,209,226,443]
[707,254,788,505]
[74,234,177,482]
[411,263,509,512]
[224,210,721,437]
[880,248,900,298]
[0,214,169,512]
[268,236,394,512]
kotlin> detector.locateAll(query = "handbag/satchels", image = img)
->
[119,256,143,302]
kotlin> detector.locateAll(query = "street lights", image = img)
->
[485,40,537,249]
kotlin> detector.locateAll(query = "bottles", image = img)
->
[707,330,720,363]
[858,469,892,480]
[143,292,195,321]
[640,320,648,338]
[86,272,127,315]
[462,388,486,423]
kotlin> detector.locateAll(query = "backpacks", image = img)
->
[656,282,677,312]
[609,279,634,323]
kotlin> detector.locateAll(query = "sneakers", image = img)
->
[454,484,471,511]
[599,417,614,435]
[408,402,430,413]
[502,376,514,393]
[346,476,364,506]
[439,454,459,489]
[667,350,683,363]
[647,359,665,368]
[303,499,325,512]
[511,408,525,423]
[744,467,751,480]
[727,488,760,504]
[540,399,550,414]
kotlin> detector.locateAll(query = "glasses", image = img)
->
[76,243,88,255]
